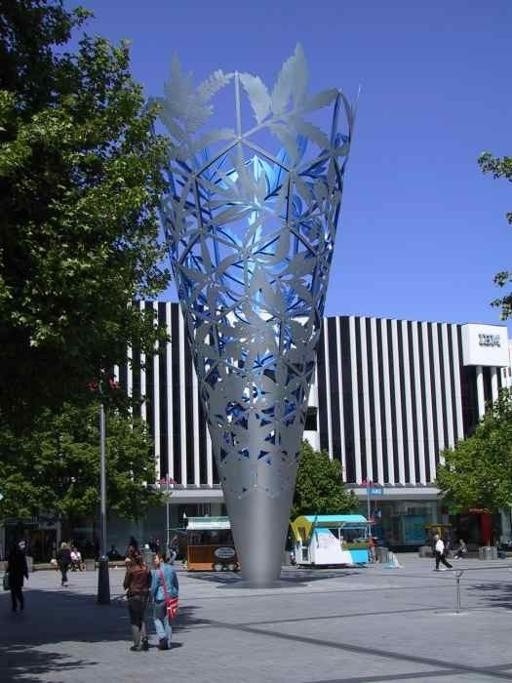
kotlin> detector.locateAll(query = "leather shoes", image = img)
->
[131,637,148,650]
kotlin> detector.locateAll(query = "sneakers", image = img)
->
[160,639,171,649]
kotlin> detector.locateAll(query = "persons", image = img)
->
[56,542,71,587]
[107,536,180,564]
[151,552,178,649]
[70,547,83,571]
[454,539,468,559]
[433,535,454,571]
[124,556,152,651]
[5,544,28,612]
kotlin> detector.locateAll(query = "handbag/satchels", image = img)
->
[4,575,11,590]
[166,597,177,619]
